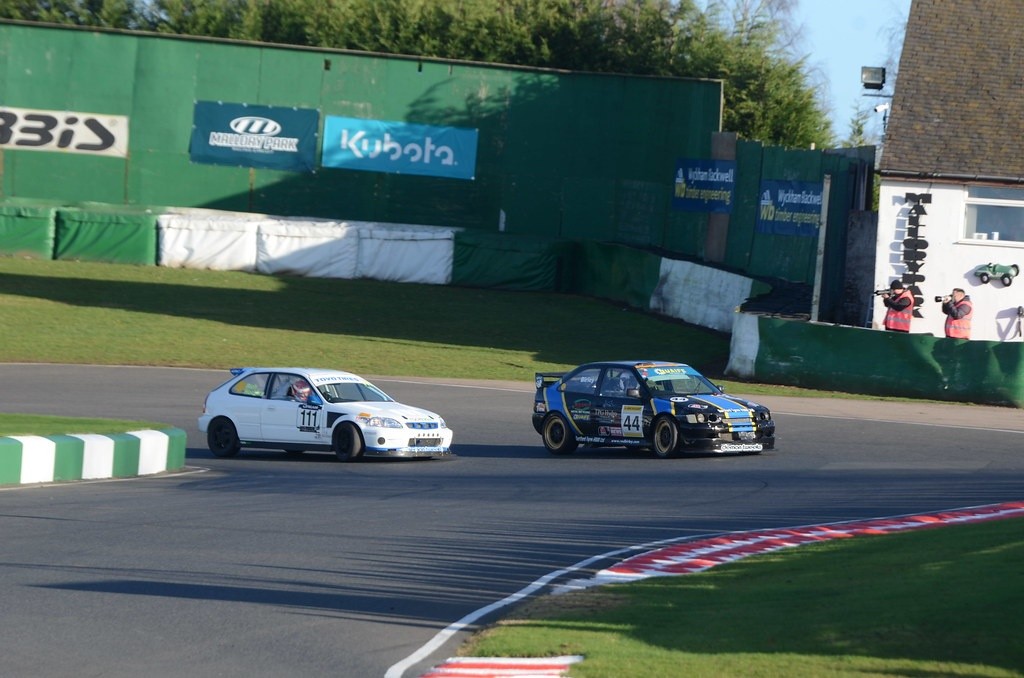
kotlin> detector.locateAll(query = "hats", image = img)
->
[890,280,903,290]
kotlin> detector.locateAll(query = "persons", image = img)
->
[942,289,972,341]
[291,381,310,403]
[882,280,914,333]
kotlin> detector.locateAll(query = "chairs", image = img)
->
[293,381,311,402]
[626,388,641,398]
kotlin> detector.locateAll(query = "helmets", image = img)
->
[291,381,311,403]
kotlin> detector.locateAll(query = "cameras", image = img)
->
[874,289,893,297]
[935,295,954,304]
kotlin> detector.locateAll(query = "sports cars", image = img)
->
[974,263,1020,287]
[195,366,453,463]
[532,359,777,458]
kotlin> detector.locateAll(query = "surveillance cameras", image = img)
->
[874,104,889,113]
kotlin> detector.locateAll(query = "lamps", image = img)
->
[860,65,895,99]
